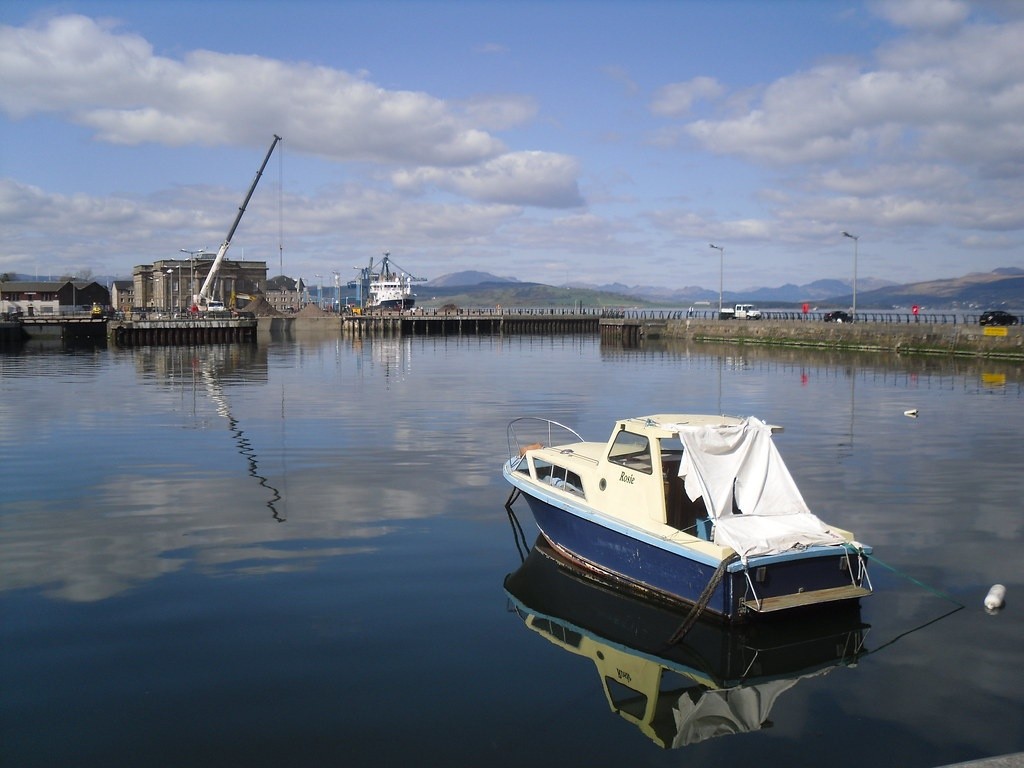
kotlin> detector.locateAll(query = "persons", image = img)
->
[688,306,693,317]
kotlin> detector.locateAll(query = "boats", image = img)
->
[501,413,877,628]
[502,527,877,751]
[346,250,428,312]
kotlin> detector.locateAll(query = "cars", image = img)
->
[823,310,852,325]
[979,310,1019,327]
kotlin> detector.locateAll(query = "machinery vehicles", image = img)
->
[185,132,285,317]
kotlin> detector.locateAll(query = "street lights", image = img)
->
[842,231,858,324]
[709,244,724,318]
[180,249,204,313]
[161,264,179,314]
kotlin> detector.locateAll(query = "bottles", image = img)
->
[984,583,1006,609]
[904,409,919,415]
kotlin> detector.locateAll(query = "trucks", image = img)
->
[720,303,762,321]
